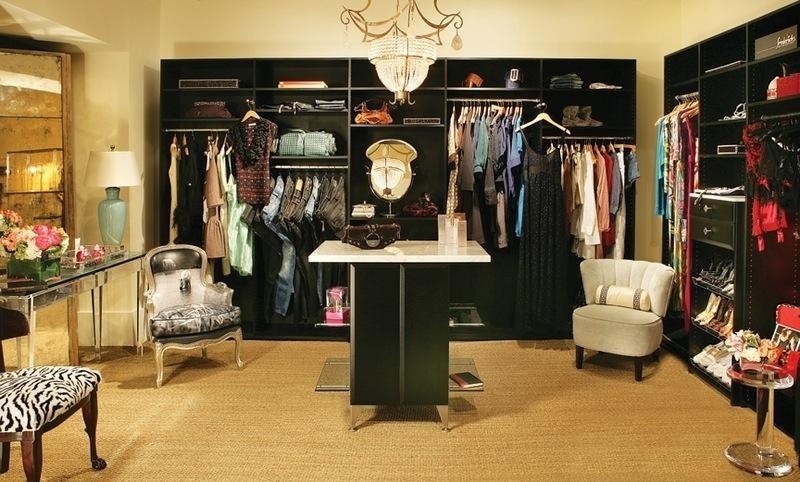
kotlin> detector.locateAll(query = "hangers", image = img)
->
[655,92,700,129]
[748,115,798,145]
[446,92,638,160]
[165,99,348,187]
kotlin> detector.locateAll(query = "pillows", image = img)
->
[594,285,652,311]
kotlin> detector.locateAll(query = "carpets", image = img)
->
[312,356,489,394]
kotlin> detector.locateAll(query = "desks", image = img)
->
[723,360,796,480]
[1,247,150,374]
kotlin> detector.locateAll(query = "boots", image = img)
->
[562,105,590,127]
[578,105,605,127]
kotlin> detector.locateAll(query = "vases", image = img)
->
[7,257,63,287]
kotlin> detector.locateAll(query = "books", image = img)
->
[450,369,484,389]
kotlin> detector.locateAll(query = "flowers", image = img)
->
[0,223,70,259]
[725,327,778,371]
[0,209,24,237]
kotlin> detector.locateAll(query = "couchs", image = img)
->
[568,256,679,383]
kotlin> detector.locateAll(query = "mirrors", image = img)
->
[0,32,83,371]
[363,138,419,222]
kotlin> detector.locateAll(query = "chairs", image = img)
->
[138,242,248,389]
[0,307,108,480]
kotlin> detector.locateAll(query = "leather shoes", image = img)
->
[699,256,735,297]
[693,293,735,338]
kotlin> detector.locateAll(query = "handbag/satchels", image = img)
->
[352,200,376,218]
[402,191,438,217]
[277,128,305,156]
[341,224,401,249]
[304,130,338,157]
[184,101,231,119]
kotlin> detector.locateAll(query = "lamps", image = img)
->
[337,0,467,109]
[80,142,147,252]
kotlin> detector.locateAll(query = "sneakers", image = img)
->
[692,339,731,385]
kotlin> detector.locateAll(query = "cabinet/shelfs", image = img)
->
[158,58,641,345]
[659,0,799,443]
[5,148,64,232]
[305,237,496,435]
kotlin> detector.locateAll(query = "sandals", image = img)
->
[718,115,738,121]
[733,102,746,119]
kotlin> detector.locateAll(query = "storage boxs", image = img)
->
[753,24,798,61]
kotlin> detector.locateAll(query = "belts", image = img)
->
[504,68,529,88]
[461,72,487,87]
